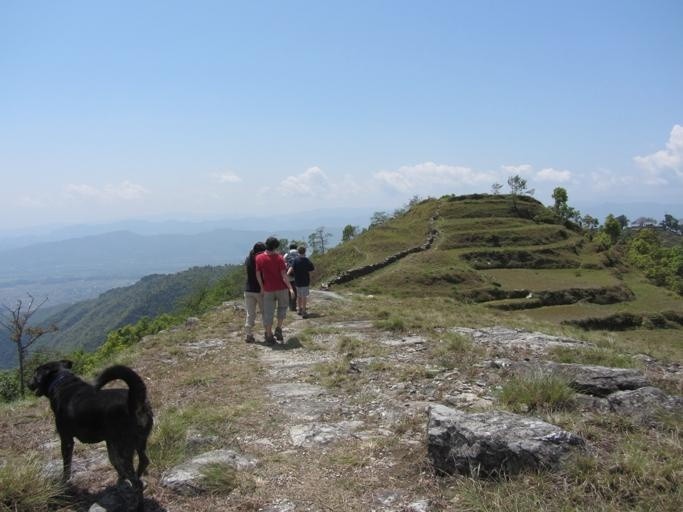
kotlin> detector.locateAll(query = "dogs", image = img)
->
[28,360,153,512]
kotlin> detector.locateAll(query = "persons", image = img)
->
[243,241,265,344]
[256,237,295,343]
[286,246,314,317]
[282,243,302,311]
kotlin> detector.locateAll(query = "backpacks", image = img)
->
[286,251,298,276]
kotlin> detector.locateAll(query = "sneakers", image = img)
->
[297,308,307,317]
[247,327,283,346]
